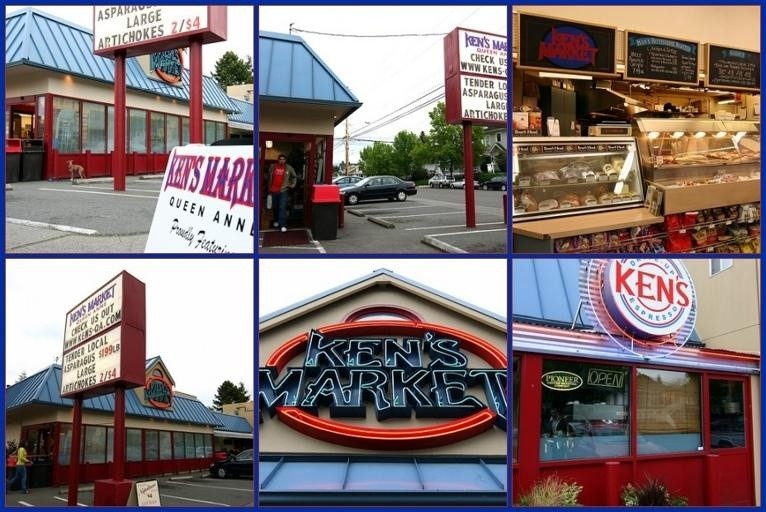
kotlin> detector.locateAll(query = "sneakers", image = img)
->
[273,222,287,233]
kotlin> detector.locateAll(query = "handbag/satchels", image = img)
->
[266,194,272,209]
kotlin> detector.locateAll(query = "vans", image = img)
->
[427,175,455,188]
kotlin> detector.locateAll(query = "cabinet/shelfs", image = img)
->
[512,116,762,254]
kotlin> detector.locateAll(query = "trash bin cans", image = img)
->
[311,184,341,241]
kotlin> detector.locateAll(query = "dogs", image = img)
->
[549,408,575,450]
[65,160,87,181]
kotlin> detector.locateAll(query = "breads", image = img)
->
[512,156,641,214]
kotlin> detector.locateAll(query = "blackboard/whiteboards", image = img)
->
[519,12,617,78]
[627,30,701,89]
[706,44,762,93]
[136,480,161,507]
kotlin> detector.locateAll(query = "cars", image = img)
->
[332,174,417,205]
[449,177,480,189]
[562,414,627,436]
[481,175,508,192]
[209,448,254,481]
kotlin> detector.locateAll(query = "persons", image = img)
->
[542,408,567,458]
[7,440,33,494]
[267,154,298,232]
